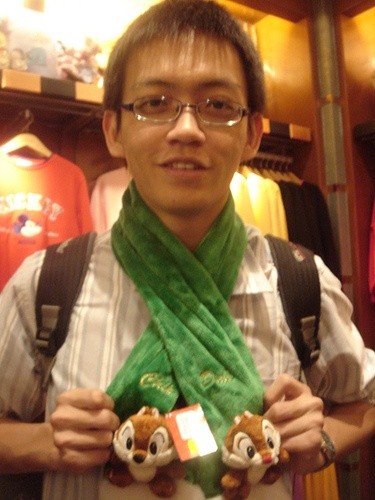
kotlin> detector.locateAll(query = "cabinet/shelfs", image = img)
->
[0,0,313,283]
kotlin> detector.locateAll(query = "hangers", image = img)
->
[240,152,298,181]
[0,110,53,163]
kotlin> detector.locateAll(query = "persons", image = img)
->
[0,0,375,500]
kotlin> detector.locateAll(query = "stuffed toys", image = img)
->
[102,406,178,497]
[221,411,289,500]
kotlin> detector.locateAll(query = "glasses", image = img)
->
[109,95,256,127]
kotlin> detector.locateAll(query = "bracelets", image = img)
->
[314,430,336,473]
